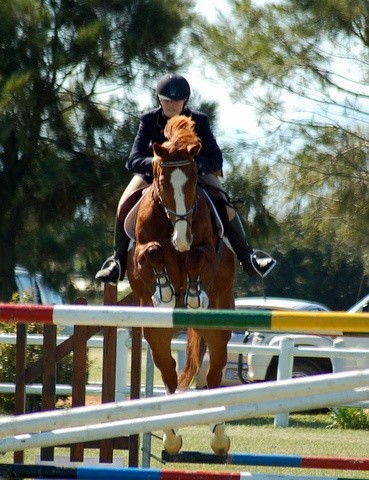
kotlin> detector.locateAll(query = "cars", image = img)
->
[197,296,369,415]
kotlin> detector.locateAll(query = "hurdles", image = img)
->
[0,280,369,480]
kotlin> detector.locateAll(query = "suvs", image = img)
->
[14,267,73,337]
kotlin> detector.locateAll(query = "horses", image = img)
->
[126,114,236,455]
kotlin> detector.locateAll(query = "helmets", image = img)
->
[156,73,191,100]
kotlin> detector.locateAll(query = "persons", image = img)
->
[95,74,276,281]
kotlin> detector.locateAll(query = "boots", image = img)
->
[95,214,130,282]
[226,211,273,276]
